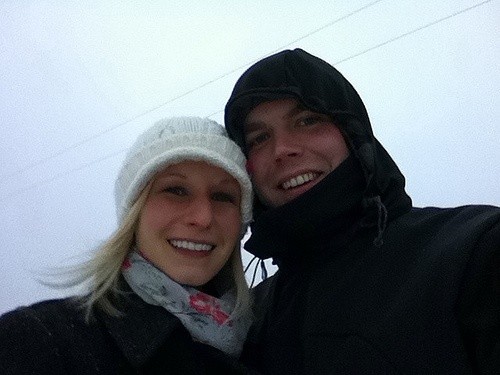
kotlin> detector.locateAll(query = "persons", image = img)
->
[223,47,500,375]
[0,119,253,375]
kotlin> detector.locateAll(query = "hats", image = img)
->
[112,115,254,239]
